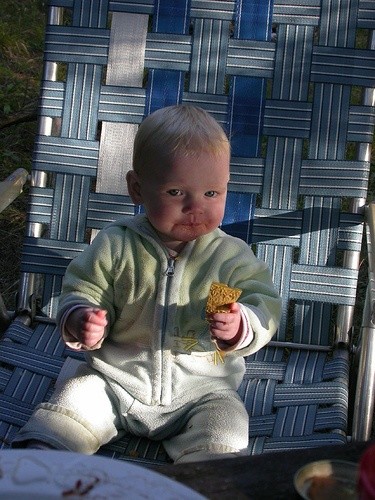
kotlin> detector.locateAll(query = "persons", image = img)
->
[12,104,287,463]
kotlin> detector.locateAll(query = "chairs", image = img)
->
[0,1,375,478]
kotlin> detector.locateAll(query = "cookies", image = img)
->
[204,282,243,322]
